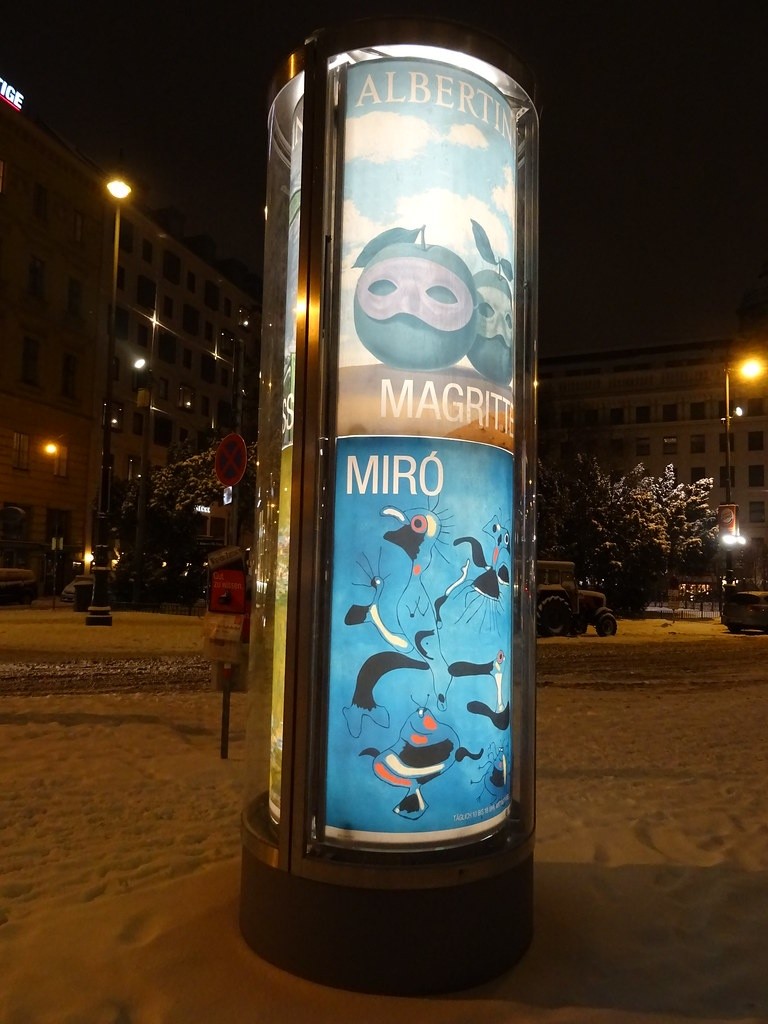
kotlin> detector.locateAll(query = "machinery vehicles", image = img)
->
[514,560,617,637]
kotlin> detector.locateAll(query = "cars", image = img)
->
[60,574,95,603]
[721,591,768,634]
[690,590,719,603]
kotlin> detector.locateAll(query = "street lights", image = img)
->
[42,441,60,609]
[221,338,246,758]
[724,356,768,593]
[85,175,134,624]
